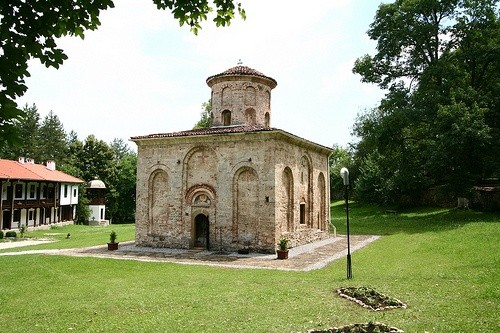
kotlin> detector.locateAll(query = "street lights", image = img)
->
[340,167,352,280]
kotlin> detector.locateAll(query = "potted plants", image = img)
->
[277,238,290,259]
[107,232,119,250]
